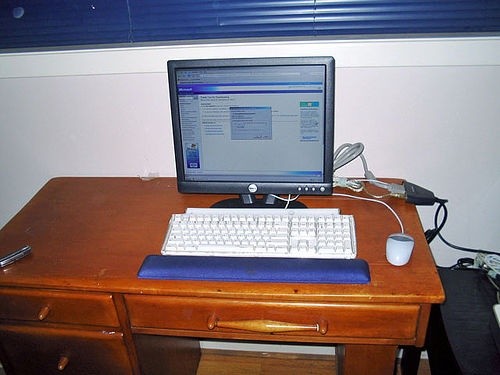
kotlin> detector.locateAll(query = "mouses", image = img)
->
[385,231,415,268]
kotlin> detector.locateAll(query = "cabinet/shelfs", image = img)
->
[122,295,420,340]
[0,288,134,375]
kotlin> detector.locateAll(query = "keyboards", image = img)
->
[161,208,358,260]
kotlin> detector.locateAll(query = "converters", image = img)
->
[404,182,435,206]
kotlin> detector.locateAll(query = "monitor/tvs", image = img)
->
[166,56,336,207]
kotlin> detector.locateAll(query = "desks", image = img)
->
[401,264,499,375]
